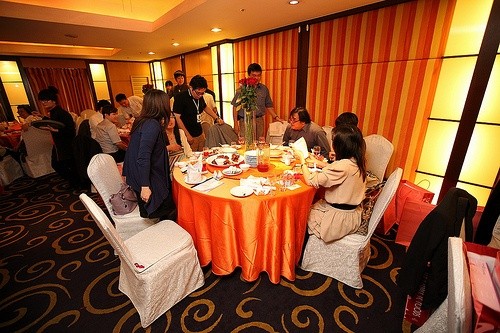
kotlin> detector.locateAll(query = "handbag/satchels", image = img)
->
[109,182,138,216]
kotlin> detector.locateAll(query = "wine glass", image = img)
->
[238,137,245,150]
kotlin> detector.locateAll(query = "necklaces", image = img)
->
[169,136,173,141]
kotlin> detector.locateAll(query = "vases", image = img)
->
[245,108,256,150]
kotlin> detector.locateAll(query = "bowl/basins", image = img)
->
[270,148,280,156]
[244,149,257,168]
[239,164,250,171]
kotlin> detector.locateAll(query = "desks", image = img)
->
[173,142,319,284]
[465,241,500,333]
[0,121,24,152]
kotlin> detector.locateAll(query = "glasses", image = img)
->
[194,89,205,96]
[17,110,24,114]
[288,118,300,125]
[252,74,261,77]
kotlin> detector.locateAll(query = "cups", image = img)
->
[289,140,295,148]
[231,142,236,150]
[277,176,287,192]
[267,172,277,195]
[293,166,301,181]
[202,160,207,172]
[203,147,209,158]
[259,137,265,146]
[287,173,295,187]
[329,152,336,161]
[313,146,321,158]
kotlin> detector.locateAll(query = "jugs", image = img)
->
[257,142,270,172]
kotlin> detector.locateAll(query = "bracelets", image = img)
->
[301,162,307,169]
[214,116,219,122]
[274,115,279,120]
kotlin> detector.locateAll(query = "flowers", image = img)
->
[236,78,259,111]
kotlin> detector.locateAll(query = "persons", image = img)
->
[31,89,77,188]
[329,112,358,161]
[115,93,144,119]
[95,105,128,163]
[291,125,367,242]
[142,70,224,156]
[4,105,42,163]
[282,106,331,159]
[231,63,284,143]
[121,89,178,223]
[89,99,110,140]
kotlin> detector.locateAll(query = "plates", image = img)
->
[180,144,327,198]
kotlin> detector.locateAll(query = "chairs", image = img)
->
[0,109,473,333]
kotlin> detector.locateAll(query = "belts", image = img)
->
[239,115,263,120]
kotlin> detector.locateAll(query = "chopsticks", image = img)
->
[191,177,214,189]
[182,169,188,174]
